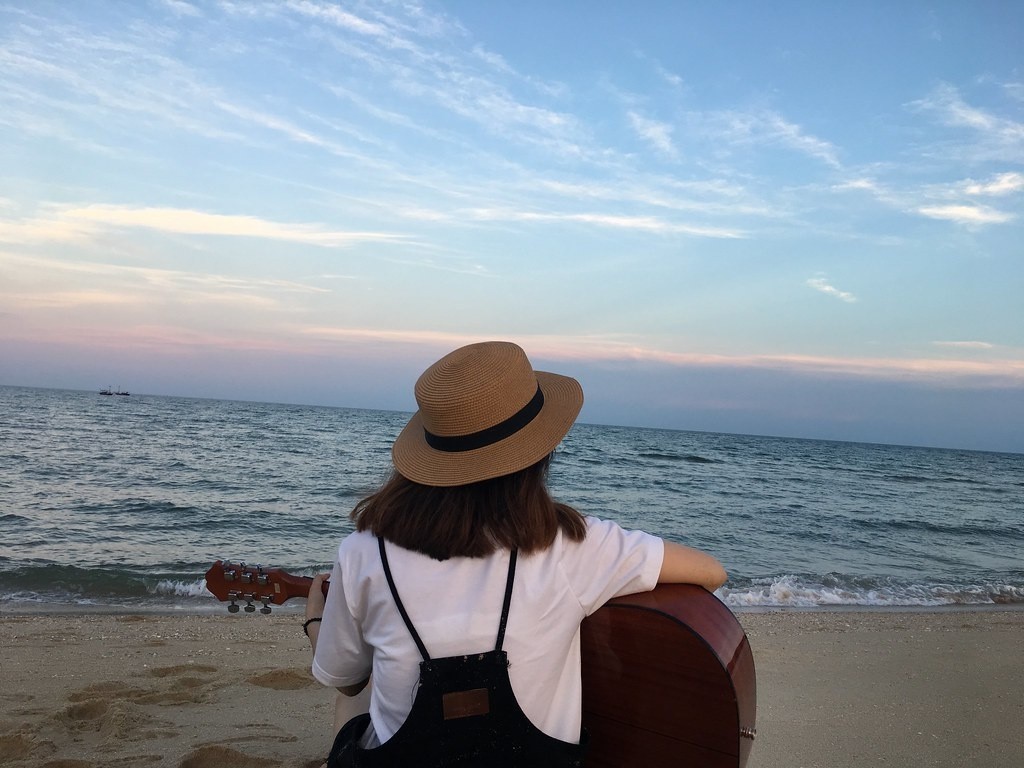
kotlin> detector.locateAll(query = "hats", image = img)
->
[392,341,584,486]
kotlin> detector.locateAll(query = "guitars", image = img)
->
[205,558,758,768]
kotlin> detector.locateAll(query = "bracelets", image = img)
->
[302,618,323,637]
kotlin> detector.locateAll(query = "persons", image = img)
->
[301,340,728,767]
